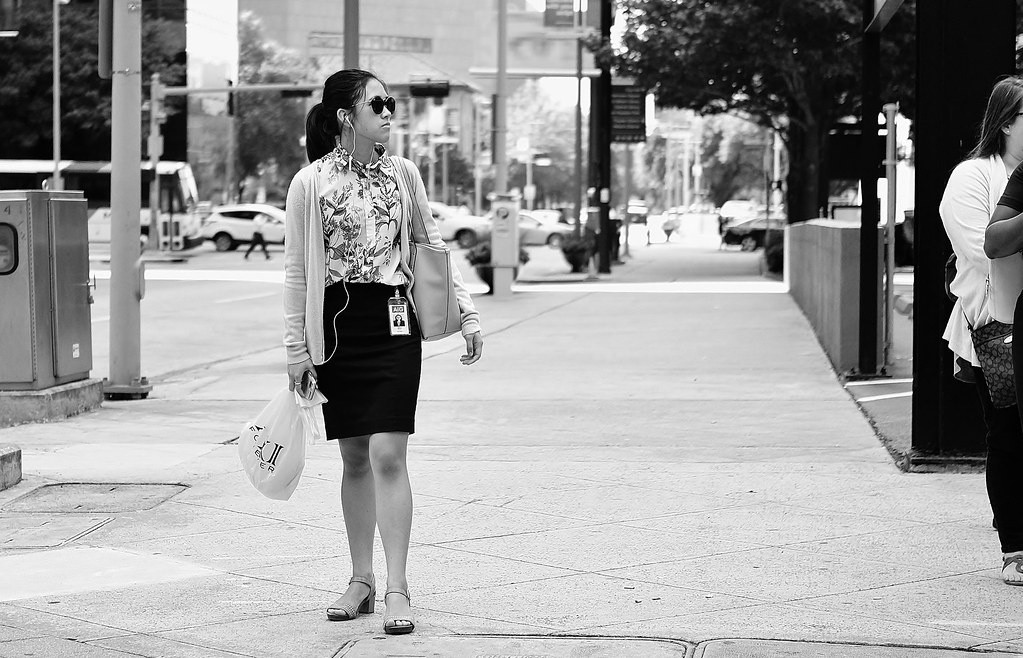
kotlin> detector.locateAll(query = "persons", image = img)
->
[935,77,1023,585]
[393,314,405,326]
[282,70,484,634]
[242,212,272,260]
[771,179,784,207]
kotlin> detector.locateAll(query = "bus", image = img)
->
[0,158,205,262]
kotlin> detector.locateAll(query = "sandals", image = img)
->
[1002,554,1023,586]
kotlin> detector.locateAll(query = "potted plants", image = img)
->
[465,240,530,294]
[559,232,599,272]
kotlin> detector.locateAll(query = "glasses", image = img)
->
[350,96,396,115]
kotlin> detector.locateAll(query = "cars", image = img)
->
[200,204,287,254]
[485,209,575,248]
[426,199,494,249]
[723,213,785,251]
[579,196,780,236]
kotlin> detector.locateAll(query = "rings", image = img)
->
[294,382,300,386]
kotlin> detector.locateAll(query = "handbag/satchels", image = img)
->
[400,156,461,342]
[970,320,1018,408]
[238,387,320,501]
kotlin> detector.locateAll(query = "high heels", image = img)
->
[383,588,415,633]
[327,574,376,620]
